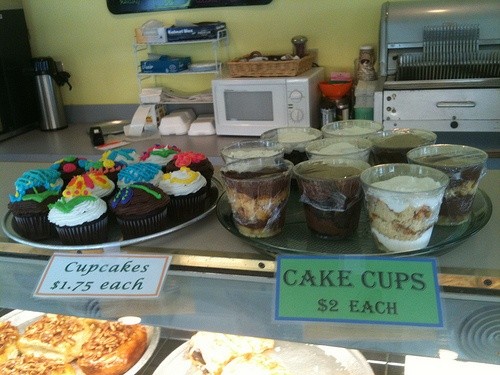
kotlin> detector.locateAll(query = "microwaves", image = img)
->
[210,66,325,138]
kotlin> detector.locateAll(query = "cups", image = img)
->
[407,144,488,226]
[305,134,375,168]
[221,140,288,165]
[373,128,440,164]
[321,119,385,137]
[360,163,450,253]
[294,159,371,242]
[220,155,294,239]
[260,125,324,191]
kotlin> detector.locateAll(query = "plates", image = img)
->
[152,330,373,375]
[0,306,159,375]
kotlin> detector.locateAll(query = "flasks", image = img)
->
[33,55,68,131]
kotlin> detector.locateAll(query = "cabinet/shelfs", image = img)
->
[132,28,230,105]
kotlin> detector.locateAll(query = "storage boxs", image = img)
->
[144,103,168,131]
[135,19,227,73]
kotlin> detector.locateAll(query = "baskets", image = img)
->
[227,49,315,77]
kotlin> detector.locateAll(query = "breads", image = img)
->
[186,331,295,375]
[0,314,148,375]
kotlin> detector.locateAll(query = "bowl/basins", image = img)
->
[319,80,352,100]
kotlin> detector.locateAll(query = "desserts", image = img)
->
[8,144,214,245]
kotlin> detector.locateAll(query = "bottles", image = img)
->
[352,44,376,91]
[337,104,350,120]
